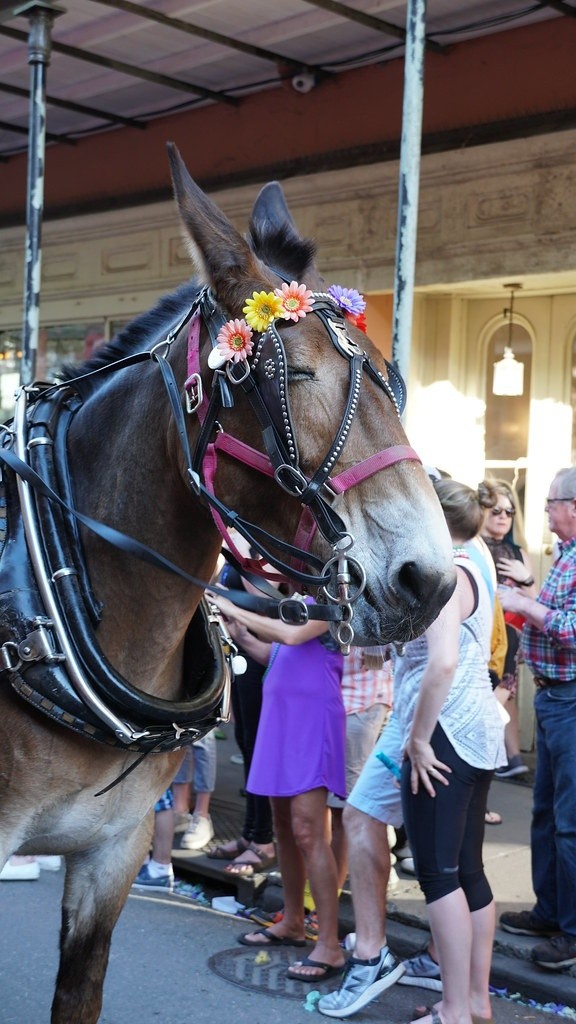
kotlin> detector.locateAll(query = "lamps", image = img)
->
[492,282,523,397]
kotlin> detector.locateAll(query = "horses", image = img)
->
[0,138,457,1024]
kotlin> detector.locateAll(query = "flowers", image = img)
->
[222,281,368,360]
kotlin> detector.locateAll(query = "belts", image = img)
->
[534,678,561,687]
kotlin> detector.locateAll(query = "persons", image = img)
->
[496,467,576,967]
[0,527,445,1018]
[397,603,506,874]
[475,478,536,779]
[393,474,508,1024]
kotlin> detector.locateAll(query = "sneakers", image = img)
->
[173,808,193,835]
[129,866,173,893]
[499,911,563,937]
[181,811,215,850]
[318,944,406,1018]
[532,931,576,970]
[494,753,529,777]
[395,950,443,993]
[250,904,322,940]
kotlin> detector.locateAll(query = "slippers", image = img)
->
[286,947,345,982]
[408,1004,495,1024]
[238,926,306,948]
[224,846,279,876]
[483,809,503,824]
[207,839,254,859]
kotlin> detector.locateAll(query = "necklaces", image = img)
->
[260,591,310,679]
[452,545,471,558]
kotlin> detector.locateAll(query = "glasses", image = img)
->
[487,507,515,518]
[546,497,576,506]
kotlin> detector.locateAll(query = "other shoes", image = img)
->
[35,855,61,870]
[0,861,40,880]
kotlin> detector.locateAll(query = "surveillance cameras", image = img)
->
[293,75,313,93]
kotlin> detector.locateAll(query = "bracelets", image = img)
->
[522,582,533,588]
